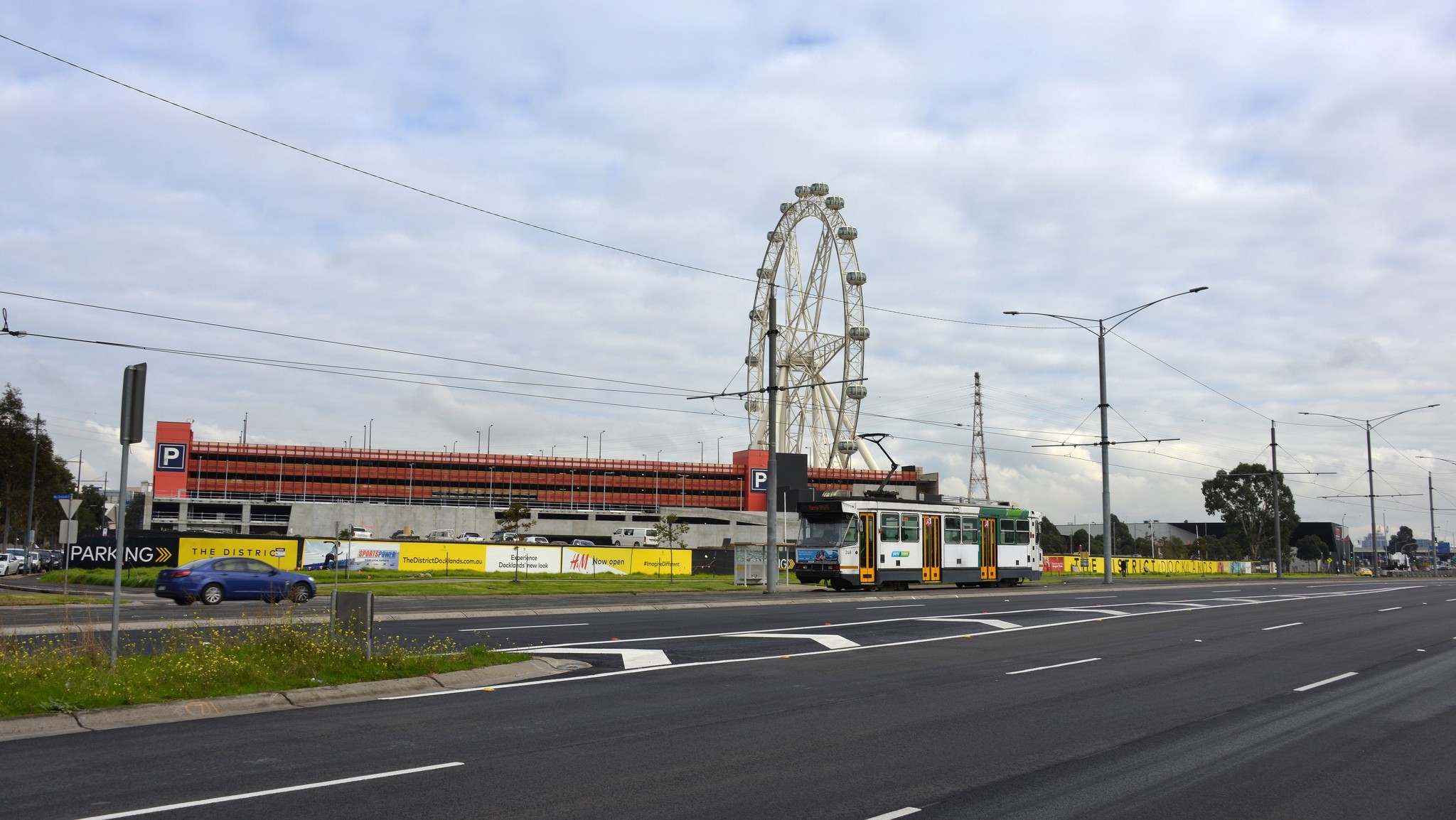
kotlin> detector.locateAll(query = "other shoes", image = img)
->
[1123,575,1126,577]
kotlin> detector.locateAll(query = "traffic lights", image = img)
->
[1413,557,1416,562]
[1435,537,1437,543]
[1350,553,1353,559]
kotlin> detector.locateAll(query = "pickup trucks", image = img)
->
[352,526,372,540]
[426,528,457,542]
[457,532,485,542]
[391,530,420,540]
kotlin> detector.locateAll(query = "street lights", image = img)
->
[1341,514,1347,575]
[1003,286,1210,584]
[1438,528,1456,558]
[193,412,743,514]
[1297,404,1442,576]
[1383,509,1388,565]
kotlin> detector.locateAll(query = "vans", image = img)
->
[490,530,520,541]
[611,527,659,547]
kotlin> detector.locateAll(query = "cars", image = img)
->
[0,548,64,577]
[1355,568,1373,577]
[523,537,549,543]
[551,541,568,544]
[1437,566,1450,571]
[570,539,596,546]
[153,557,317,605]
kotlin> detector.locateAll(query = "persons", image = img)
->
[322,541,343,571]
[1335,566,1339,575]
[814,550,825,561]
[1121,559,1126,577]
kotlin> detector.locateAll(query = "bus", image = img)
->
[795,433,1045,591]
